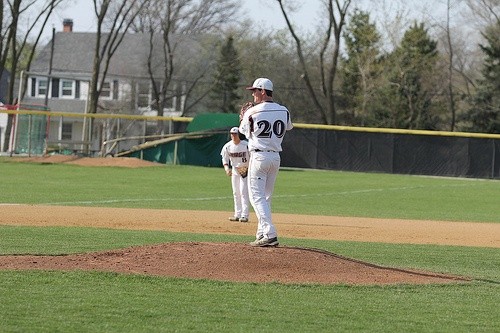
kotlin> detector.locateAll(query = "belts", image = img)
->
[255,148,279,152]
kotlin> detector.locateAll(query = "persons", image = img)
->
[220,127,250,222]
[238,78,293,247]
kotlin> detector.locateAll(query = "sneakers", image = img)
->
[255,235,280,247]
[228,216,240,221]
[239,216,249,222]
[249,234,265,246]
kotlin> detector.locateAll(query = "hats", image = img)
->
[246,77,274,92]
[229,126,240,134]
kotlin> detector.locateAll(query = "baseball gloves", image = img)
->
[235,166,248,179]
[240,102,254,119]
